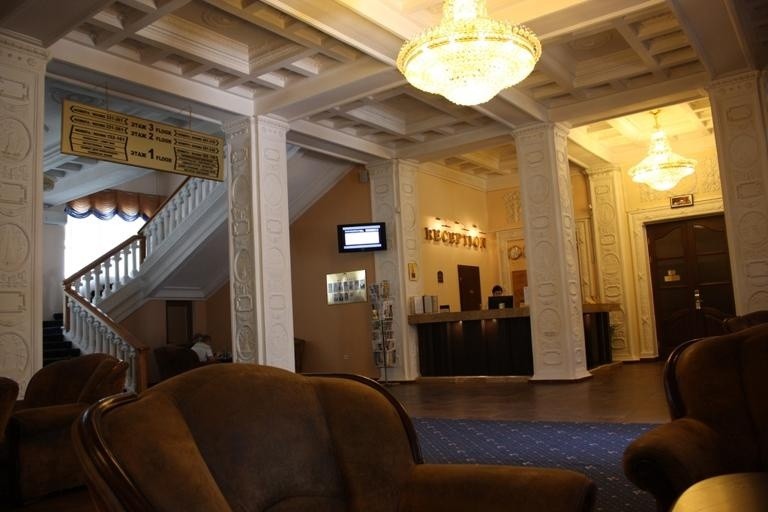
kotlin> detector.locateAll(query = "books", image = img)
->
[369,280,396,368]
[410,295,438,314]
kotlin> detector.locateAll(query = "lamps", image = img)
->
[629,109,696,193]
[393,0,545,107]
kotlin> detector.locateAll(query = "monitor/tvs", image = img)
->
[337,222,386,252]
[488,295,513,309]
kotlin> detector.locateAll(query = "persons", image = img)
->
[187,333,202,349]
[484,284,504,311]
[191,335,215,365]
